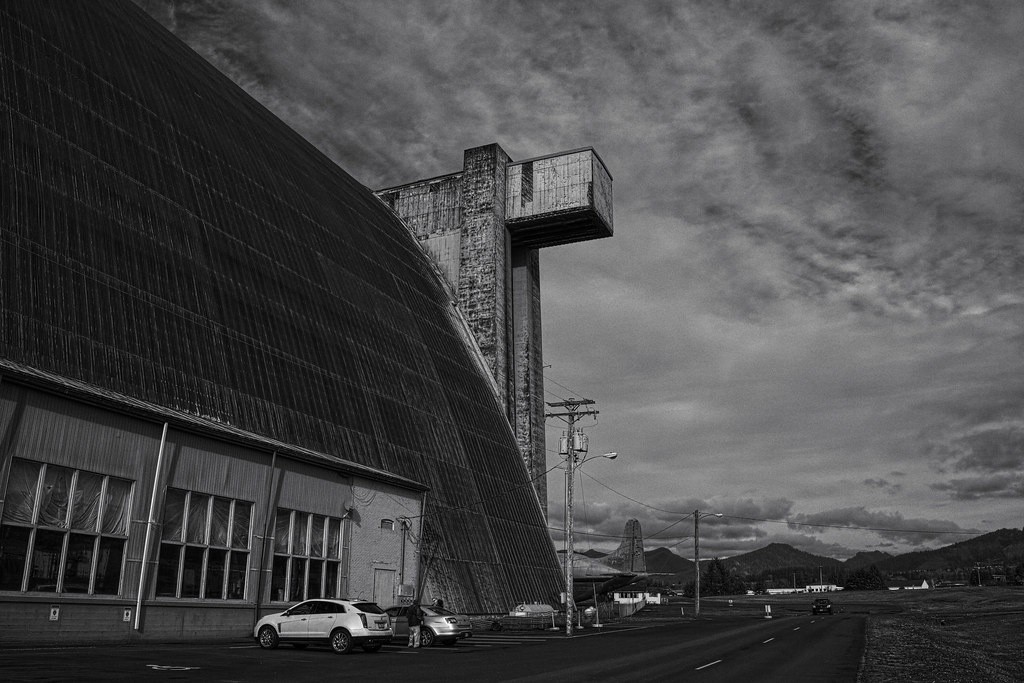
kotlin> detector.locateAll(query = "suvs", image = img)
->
[254,596,394,655]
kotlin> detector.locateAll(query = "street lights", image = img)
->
[694,510,723,619]
[565,451,618,637]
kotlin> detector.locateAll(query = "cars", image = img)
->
[384,603,474,648]
[811,598,833,615]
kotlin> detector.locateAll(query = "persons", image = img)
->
[406,600,424,648]
[432,597,444,612]
